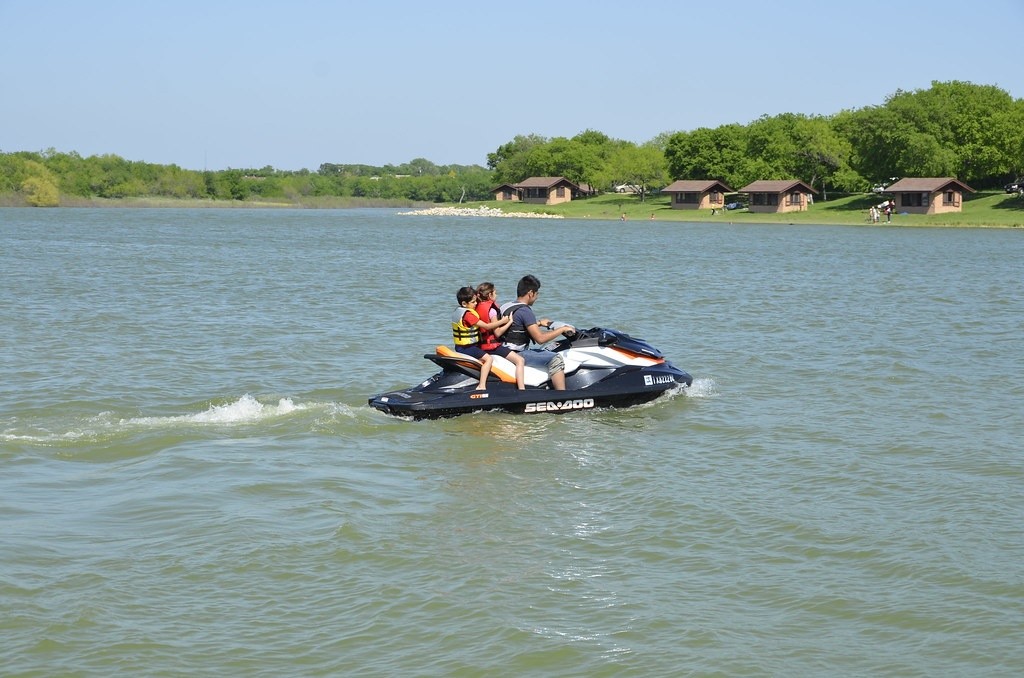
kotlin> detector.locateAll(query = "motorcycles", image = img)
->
[367,321,693,422]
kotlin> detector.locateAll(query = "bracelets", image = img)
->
[539,320,541,325]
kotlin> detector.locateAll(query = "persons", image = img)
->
[869,198,895,224]
[501,275,576,390]
[452,282,525,390]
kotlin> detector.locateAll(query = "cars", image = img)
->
[872,176,899,194]
[1004,177,1024,196]
[615,181,643,194]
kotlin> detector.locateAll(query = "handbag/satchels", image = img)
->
[884,209,888,215]
[886,208,891,212]
